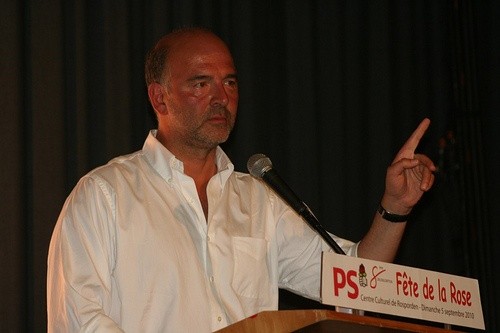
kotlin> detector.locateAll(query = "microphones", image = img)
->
[247,153,307,215]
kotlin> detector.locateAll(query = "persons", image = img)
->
[46,27,438,333]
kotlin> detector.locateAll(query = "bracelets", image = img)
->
[376,202,411,223]
